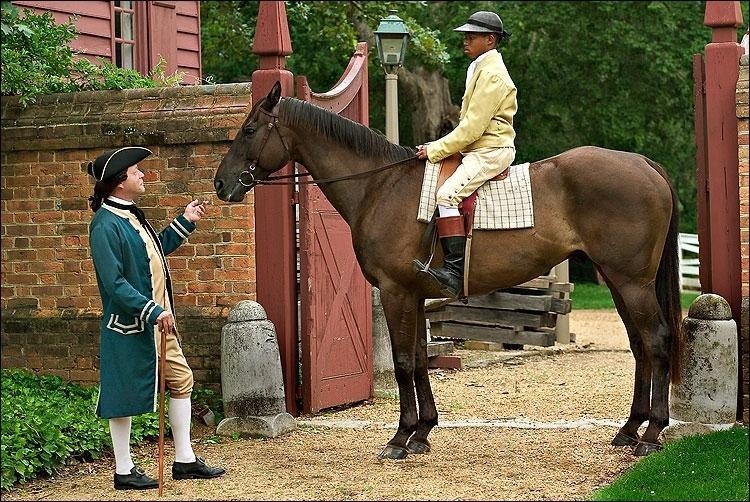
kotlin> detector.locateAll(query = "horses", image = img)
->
[214,81,683,459]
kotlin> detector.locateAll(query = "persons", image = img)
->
[86,147,224,489]
[411,11,518,299]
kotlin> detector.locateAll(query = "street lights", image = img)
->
[372,9,411,146]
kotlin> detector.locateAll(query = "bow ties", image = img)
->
[102,197,147,225]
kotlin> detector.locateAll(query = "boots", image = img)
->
[411,214,467,299]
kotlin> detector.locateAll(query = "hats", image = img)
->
[84,144,155,184]
[452,10,505,36]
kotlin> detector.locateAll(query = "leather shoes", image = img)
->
[172,457,227,481]
[113,465,166,491]
